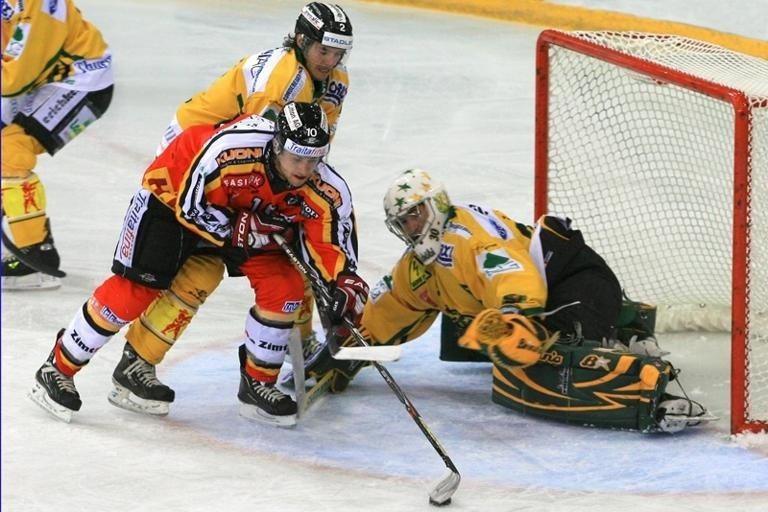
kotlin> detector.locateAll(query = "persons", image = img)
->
[0,0,113,276]
[113,1,354,402]
[281,170,687,432]
[36,102,368,416]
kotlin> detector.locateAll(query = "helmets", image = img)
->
[382,167,449,267]
[273,101,330,176]
[294,1,352,70]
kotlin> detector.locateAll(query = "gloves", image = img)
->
[328,271,369,345]
[231,207,293,254]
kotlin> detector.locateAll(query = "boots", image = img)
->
[112,342,174,402]
[285,332,322,363]
[35,328,81,411]
[236,342,296,416]
[0,216,58,276]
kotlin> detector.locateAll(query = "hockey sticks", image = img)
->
[312,284,402,361]
[273,233,460,504]
[0,211,66,277]
[289,326,335,419]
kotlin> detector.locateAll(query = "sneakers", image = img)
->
[657,392,710,432]
[592,324,661,360]
